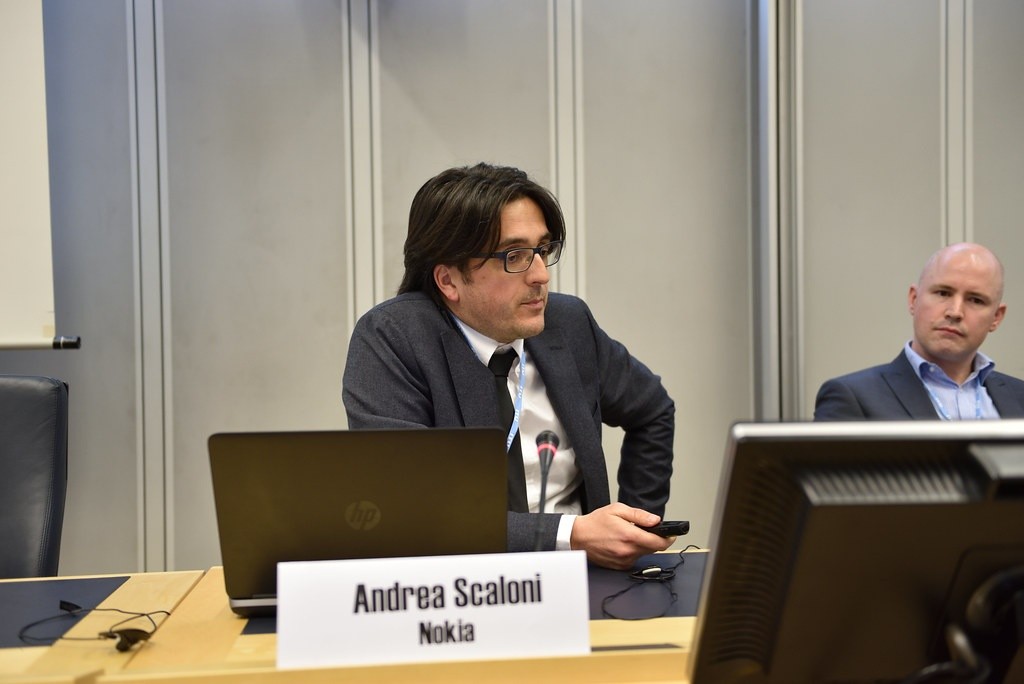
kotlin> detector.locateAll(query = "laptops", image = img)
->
[207,426,506,617]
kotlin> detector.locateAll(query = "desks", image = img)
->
[0,550,711,684]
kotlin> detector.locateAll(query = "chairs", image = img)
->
[0,374,68,578]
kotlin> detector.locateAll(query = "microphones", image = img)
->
[534,430,560,551]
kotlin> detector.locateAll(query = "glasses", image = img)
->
[471,240,563,274]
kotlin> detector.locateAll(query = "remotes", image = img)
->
[636,520,689,538]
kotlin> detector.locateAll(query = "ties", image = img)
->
[488,348,530,514]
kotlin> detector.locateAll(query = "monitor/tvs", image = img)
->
[689,418,1024,684]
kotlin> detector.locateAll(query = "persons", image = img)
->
[814,243,1023,421]
[342,162,679,570]
